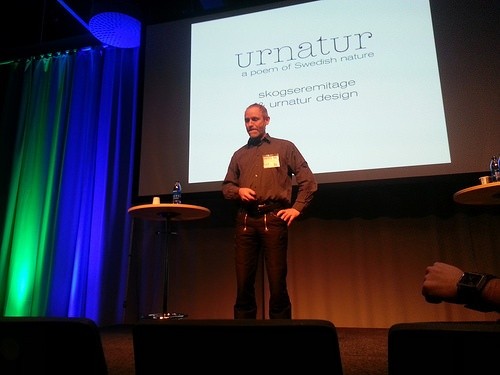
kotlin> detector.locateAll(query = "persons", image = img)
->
[422,262,500,313]
[223,103,318,319]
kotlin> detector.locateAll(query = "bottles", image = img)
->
[173,180,181,204]
[490,155,500,182]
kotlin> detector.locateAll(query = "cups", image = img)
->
[479,175,490,185]
[152,197,160,204]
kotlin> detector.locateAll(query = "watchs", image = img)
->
[456,271,496,308]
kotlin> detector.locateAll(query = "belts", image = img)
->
[239,203,288,214]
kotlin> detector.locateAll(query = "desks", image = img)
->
[453,181,500,205]
[127,203,211,319]
